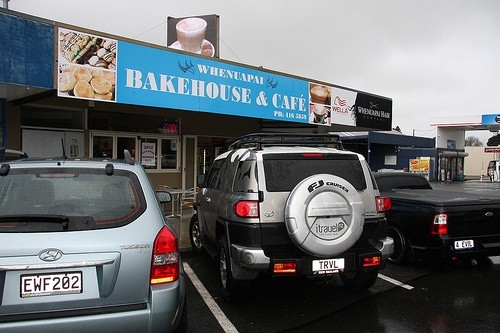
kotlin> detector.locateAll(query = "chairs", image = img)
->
[178,187,200,222]
[28,180,54,209]
[102,184,129,209]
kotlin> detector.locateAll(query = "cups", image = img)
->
[176,18,208,54]
[313,107,328,123]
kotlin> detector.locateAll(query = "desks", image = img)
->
[162,189,188,219]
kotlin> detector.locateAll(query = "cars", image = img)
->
[155,154,177,168]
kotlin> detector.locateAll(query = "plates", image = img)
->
[170,39,215,57]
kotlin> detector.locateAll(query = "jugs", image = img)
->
[70,139,79,157]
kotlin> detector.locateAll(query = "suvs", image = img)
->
[189,132,391,305]
[0,148,188,333]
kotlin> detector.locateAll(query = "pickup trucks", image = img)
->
[372,172,500,269]
